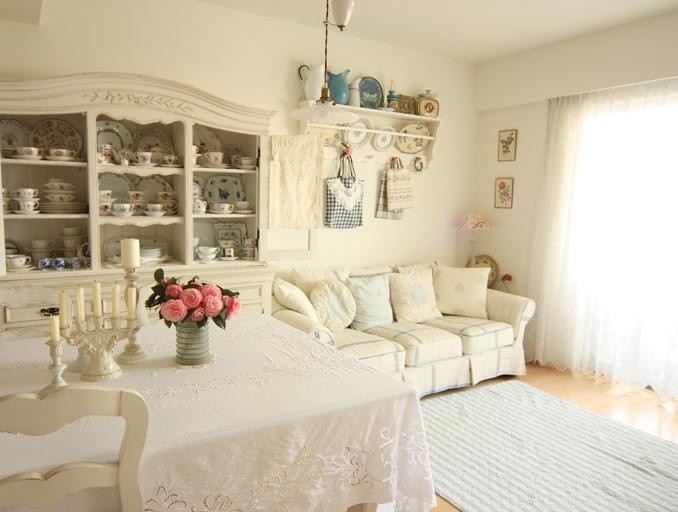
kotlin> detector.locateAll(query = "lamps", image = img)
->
[457,212,500,266]
[324,1,356,34]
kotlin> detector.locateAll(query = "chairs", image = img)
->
[1,381,149,511]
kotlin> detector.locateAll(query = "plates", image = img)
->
[30,118,84,157]
[160,163,184,168]
[235,165,257,169]
[203,175,246,209]
[241,257,256,260]
[216,227,242,248]
[12,156,44,161]
[220,145,245,169]
[240,247,257,250]
[154,199,178,204]
[131,211,146,216]
[46,156,78,162]
[111,212,134,217]
[6,266,36,273]
[99,198,117,204]
[166,211,178,215]
[39,202,89,213]
[193,181,204,199]
[57,235,87,239]
[207,210,235,214]
[374,126,397,152]
[143,211,167,217]
[13,210,41,214]
[357,76,385,109]
[193,176,206,198]
[0,118,33,149]
[124,200,149,204]
[396,124,430,154]
[219,256,240,261]
[13,198,40,201]
[344,117,374,150]
[98,172,135,206]
[140,247,162,258]
[2,198,13,201]
[202,163,229,169]
[233,210,255,214]
[132,174,174,210]
[193,126,224,164]
[3,210,11,214]
[193,221,217,248]
[26,249,56,253]
[131,162,159,167]
[466,255,498,289]
[322,122,344,148]
[133,126,175,163]
[96,121,133,152]
[103,235,129,258]
[58,248,88,251]
[213,223,247,252]
[143,142,175,166]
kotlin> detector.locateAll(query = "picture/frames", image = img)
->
[498,127,518,164]
[493,175,515,211]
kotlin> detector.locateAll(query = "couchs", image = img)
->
[272,257,537,401]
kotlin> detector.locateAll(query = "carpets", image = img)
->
[420,376,678,512]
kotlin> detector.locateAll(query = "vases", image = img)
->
[173,317,211,368]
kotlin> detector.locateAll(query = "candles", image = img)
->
[73,284,87,325]
[110,283,119,319]
[126,285,138,320]
[120,238,141,270]
[91,281,103,318]
[58,291,69,329]
[48,314,62,343]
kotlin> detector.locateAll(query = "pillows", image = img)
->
[430,262,492,322]
[309,276,358,333]
[387,267,444,325]
[271,276,321,321]
[346,272,393,332]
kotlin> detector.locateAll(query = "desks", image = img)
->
[0,304,440,512]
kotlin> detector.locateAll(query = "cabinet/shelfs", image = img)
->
[297,99,444,171]
[1,71,278,335]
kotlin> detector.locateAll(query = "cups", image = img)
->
[240,157,256,166]
[146,203,167,212]
[243,238,256,248]
[16,147,43,155]
[196,254,218,261]
[218,240,240,248]
[63,252,78,257]
[163,155,178,165]
[62,224,87,235]
[2,202,10,210]
[61,239,86,248]
[205,152,225,163]
[49,148,77,157]
[163,204,178,209]
[6,255,31,267]
[100,190,113,199]
[99,204,111,216]
[38,258,49,270]
[2,189,9,196]
[135,152,152,163]
[212,204,235,210]
[32,240,56,249]
[19,201,39,210]
[243,252,255,256]
[198,246,220,253]
[127,190,149,200]
[158,193,177,200]
[53,258,65,270]
[131,204,145,211]
[18,188,39,198]
[32,254,49,262]
[236,202,250,209]
[112,203,134,212]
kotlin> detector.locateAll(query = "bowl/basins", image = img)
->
[0,146,18,159]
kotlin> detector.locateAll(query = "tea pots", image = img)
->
[113,146,135,163]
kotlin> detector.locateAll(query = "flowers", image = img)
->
[143,268,241,331]
